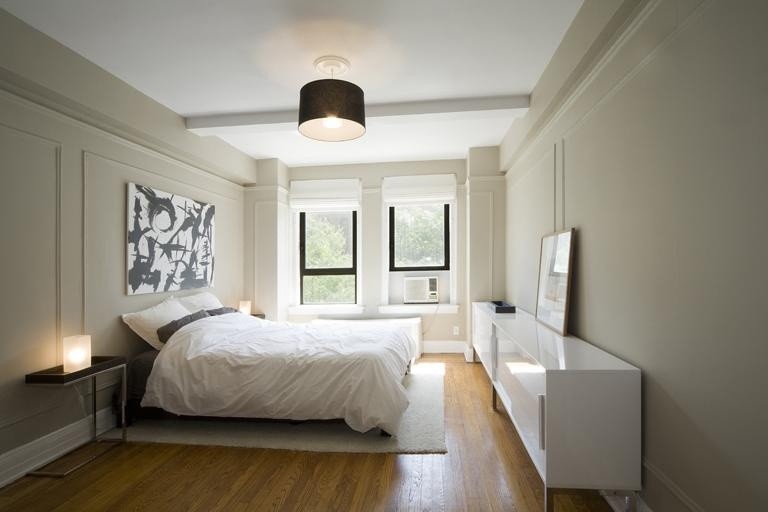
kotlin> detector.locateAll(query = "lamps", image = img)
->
[239,297,251,316]
[297,56,366,144]
[63,333,92,376]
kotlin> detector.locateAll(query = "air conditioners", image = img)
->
[402,276,438,305]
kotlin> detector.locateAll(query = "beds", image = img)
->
[126,321,421,437]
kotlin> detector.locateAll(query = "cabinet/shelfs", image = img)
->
[468,300,640,512]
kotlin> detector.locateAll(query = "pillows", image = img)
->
[123,295,190,351]
[179,290,222,313]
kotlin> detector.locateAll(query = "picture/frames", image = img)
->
[534,227,577,336]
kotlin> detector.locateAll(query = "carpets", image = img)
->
[105,360,447,455]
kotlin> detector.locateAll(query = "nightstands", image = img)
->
[250,310,265,319]
[21,354,126,476]
